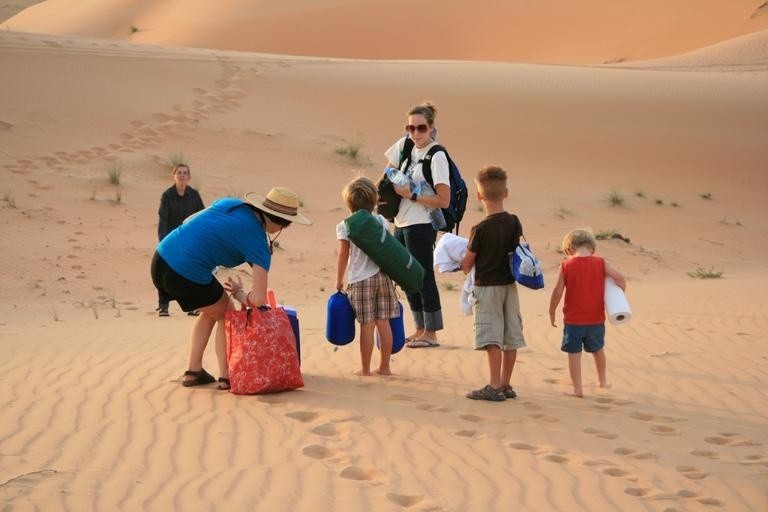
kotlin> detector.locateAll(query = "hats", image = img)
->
[244,187,312,225]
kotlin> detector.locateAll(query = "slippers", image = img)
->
[405,338,440,348]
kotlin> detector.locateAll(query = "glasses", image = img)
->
[406,125,427,134]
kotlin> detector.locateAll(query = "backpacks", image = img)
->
[398,138,467,235]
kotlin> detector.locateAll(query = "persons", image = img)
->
[457,165,543,401]
[150,186,312,391]
[383,101,468,348]
[334,177,400,376]
[157,164,205,316]
[548,228,631,398]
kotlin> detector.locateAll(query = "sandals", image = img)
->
[156,305,169,317]
[182,369,215,386]
[218,378,231,390]
[467,385,515,401]
[188,311,200,316]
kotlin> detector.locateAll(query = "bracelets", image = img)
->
[411,192,417,201]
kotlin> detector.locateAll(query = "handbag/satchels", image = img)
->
[377,301,404,354]
[246,307,300,368]
[508,244,544,289]
[326,289,355,345]
[344,208,425,294]
[224,291,304,395]
[377,173,401,223]
